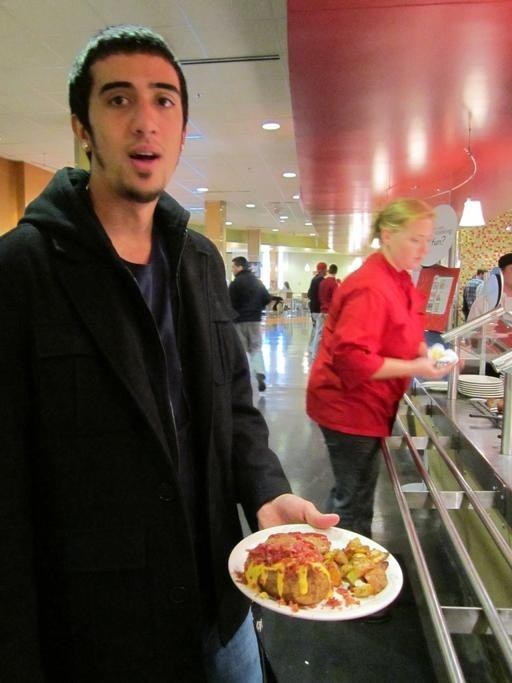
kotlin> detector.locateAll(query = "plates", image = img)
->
[457,374,506,399]
[227,524,404,622]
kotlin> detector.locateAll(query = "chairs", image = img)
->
[301,292,308,309]
[285,292,293,309]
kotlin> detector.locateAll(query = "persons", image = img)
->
[0,26,340,683]
[307,263,341,360]
[270,280,292,310]
[464,253,512,348]
[307,198,452,623]
[229,257,270,391]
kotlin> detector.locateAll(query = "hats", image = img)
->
[315,262,327,273]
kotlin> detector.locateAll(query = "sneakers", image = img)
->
[364,609,393,623]
[256,374,266,392]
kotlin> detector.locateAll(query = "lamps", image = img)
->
[458,110,487,227]
